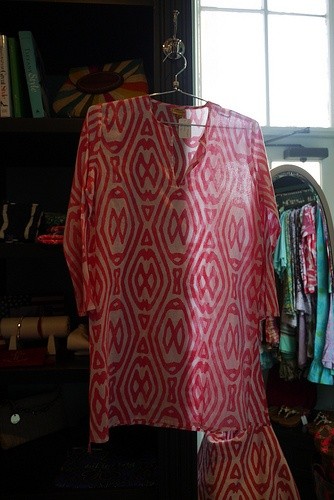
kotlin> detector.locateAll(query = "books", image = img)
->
[0,31,48,123]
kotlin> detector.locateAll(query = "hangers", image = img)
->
[151,51,260,131]
[276,189,318,210]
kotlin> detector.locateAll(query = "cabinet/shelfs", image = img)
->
[1,0,196,393]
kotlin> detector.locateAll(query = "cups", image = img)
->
[0,202,44,305]
[36,226,65,253]
